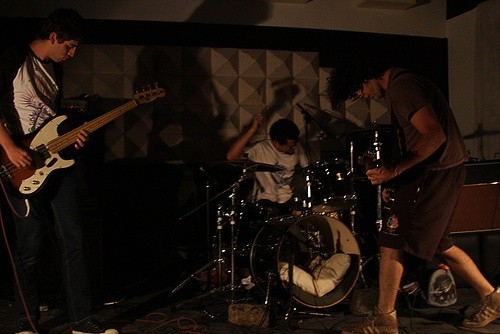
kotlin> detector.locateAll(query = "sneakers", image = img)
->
[462,287,500,328]
[72,318,119,334]
[15,319,38,334]
[342,306,398,334]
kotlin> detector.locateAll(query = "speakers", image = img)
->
[5,113,105,302]
[448,158,500,234]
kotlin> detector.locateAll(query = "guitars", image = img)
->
[0,82,166,201]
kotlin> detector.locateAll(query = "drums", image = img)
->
[221,206,262,269]
[288,200,324,217]
[250,215,363,309]
[247,199,289,230]
[308,192,361,224]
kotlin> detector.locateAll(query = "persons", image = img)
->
[227,111,312,210]
[328,54,500,334]
[0,7,118,334]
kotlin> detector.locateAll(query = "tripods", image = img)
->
[166,173,332,334]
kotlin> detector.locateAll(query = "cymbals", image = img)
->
[326,149,361,186]
[343,127,393,136]
[225,159,285,172]
[290,172,329,202]
